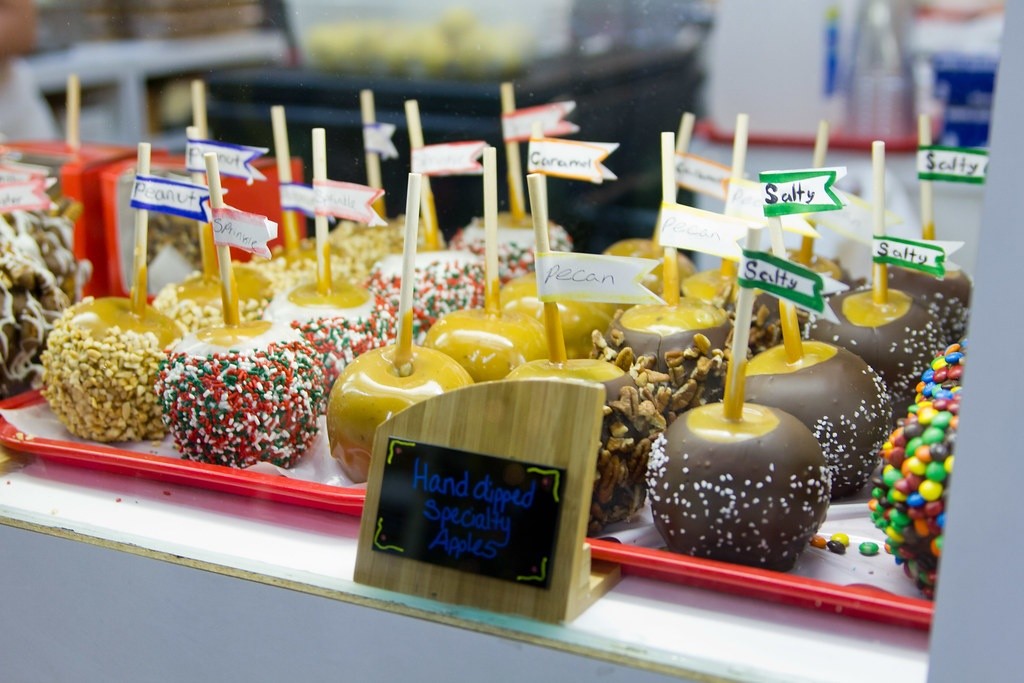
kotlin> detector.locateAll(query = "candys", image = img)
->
[40,212,971,598]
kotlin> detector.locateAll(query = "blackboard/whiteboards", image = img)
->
[371,436,568,592]
